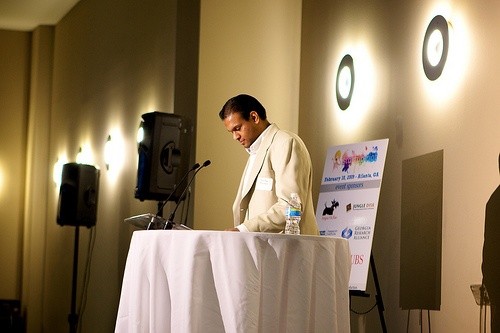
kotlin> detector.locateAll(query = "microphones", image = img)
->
[147,160,211,230]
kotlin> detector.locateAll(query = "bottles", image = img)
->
[284,192,301,235]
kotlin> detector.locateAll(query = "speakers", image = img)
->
[57,163,99,229]
[134,111,187,205]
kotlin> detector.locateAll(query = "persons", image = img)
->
[219,93,319,235]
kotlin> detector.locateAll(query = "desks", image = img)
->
[112,230,352,332]
